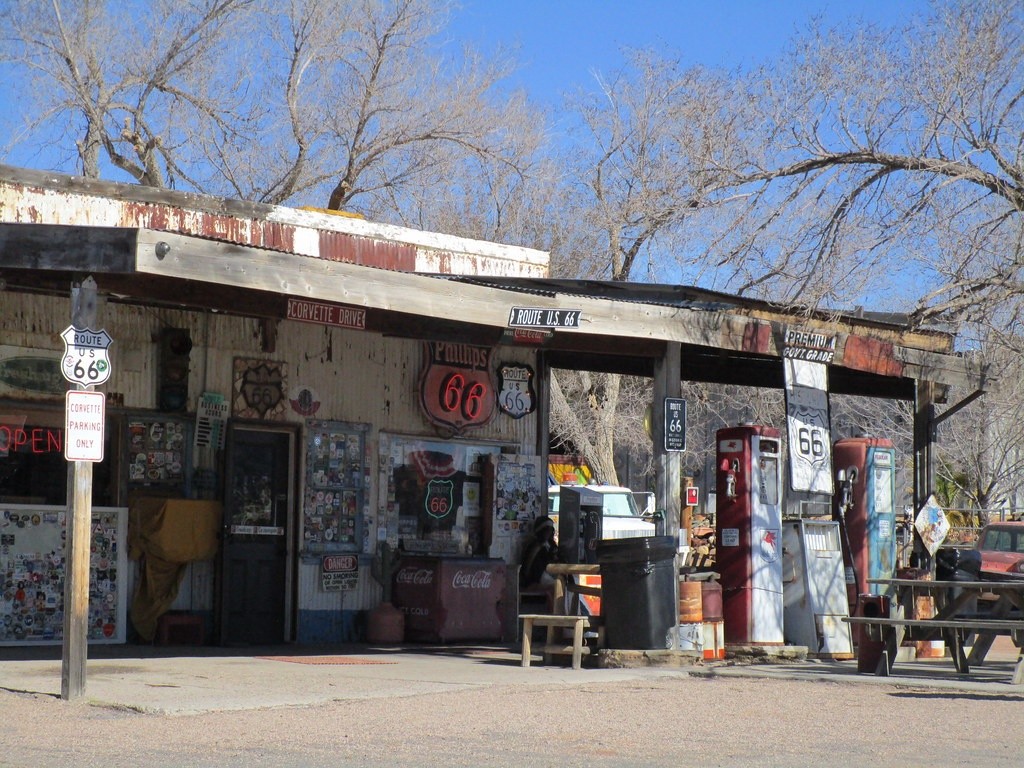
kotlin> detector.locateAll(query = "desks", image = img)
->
[865,578,1024,686]
[542,563,600,665]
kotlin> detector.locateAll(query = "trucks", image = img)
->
[548,483,657,538]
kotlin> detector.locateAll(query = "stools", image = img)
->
[157,614,205,646]
[518,613,603,670]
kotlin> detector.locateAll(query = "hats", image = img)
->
[533,515,554,534]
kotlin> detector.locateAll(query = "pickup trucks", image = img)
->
[939,520,1024,580]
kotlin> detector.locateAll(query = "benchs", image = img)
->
[840,616,1024,648]
[919,617,1024,642]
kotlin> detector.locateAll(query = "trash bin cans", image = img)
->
[596,534,681,650]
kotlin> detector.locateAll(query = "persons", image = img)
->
[520,516,592,646]
